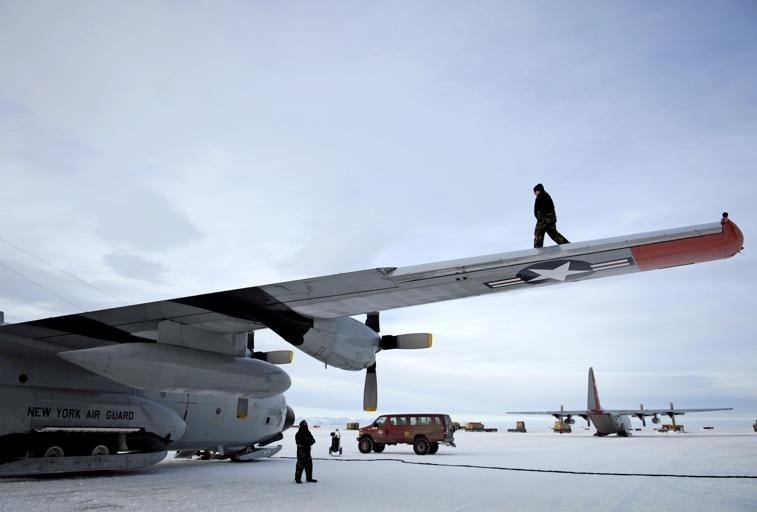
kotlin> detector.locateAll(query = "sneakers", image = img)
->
[295,479,317,483]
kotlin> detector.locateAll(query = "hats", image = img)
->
[534,183,544,192]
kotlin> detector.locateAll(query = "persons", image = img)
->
[533,183,570,247]
[295,420,317,483]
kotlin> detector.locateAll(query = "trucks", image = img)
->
[507,421,526,432]
[464,422,484,432]
[554,421,571,433]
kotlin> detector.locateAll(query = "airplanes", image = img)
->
[505,367,734,437]
[0,211,745,478]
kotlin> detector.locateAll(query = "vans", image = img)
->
[356,414,456,455]
